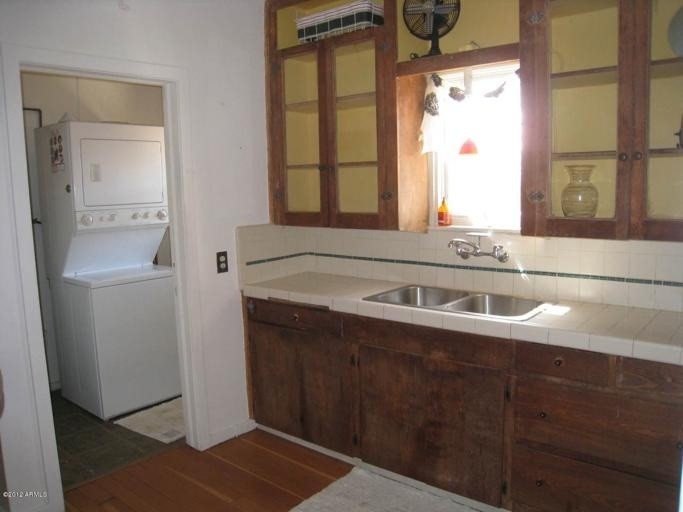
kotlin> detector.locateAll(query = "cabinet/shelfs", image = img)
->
[505,339,683,512]
[265,0,431,233]
[242,297,358,456]
[341,314,509,512]
[518,0,683,242]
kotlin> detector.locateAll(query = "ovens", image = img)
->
[68,119,170,230]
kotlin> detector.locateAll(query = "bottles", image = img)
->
[438,196,452,226]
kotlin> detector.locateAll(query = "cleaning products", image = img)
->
[438,193,451,226]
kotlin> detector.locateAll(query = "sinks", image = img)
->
[444,293,548,321]
[362,284,470,308]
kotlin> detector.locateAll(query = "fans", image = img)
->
[403,0,460,58]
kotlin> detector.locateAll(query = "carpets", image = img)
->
[52,389,163,493]
[114,395,185,444]
[289,465,482,512]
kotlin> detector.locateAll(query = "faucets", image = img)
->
[459,247,482,260]
